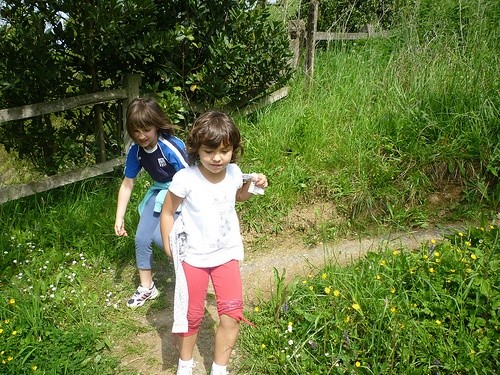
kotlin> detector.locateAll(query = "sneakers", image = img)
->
[210,369,229,375]
[126,282,158,310]
[176,363,194,375]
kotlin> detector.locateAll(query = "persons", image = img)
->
[160,112,272,375]
[111,96,194,310]
